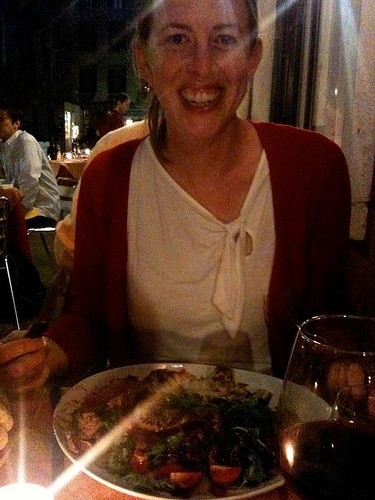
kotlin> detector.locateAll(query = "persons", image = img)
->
[0,104,62,230]
[71,122,79,152]
[0,0,375,417]
[96,91,131,137]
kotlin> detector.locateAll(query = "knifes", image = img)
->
[50,381,63,481]
[23,270,67,339]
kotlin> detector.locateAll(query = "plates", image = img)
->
[53,363,331,500]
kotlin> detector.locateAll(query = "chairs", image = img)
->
[0,196,21,330]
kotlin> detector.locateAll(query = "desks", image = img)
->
[49,158,90,217]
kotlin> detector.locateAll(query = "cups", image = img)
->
[273,314,375,500]
[330,386,375,432]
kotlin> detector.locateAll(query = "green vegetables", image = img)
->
[97,392,286,489]
